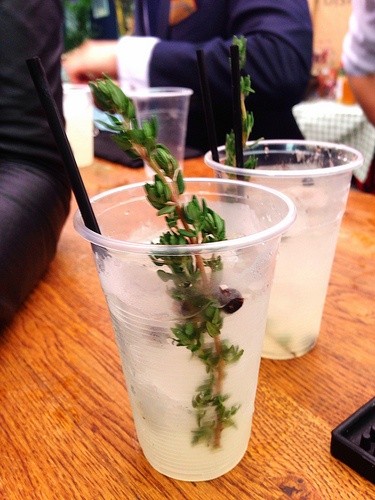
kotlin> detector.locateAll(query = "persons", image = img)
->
[0,0,72,330]
[342,0,375,129]
[65,0,314,162]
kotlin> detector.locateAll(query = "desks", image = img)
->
[0,151,375,500]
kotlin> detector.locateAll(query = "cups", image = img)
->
[131,86,193,180]
[205,139,362,360]
[73,178,296,482]
[62,84,93,168]
